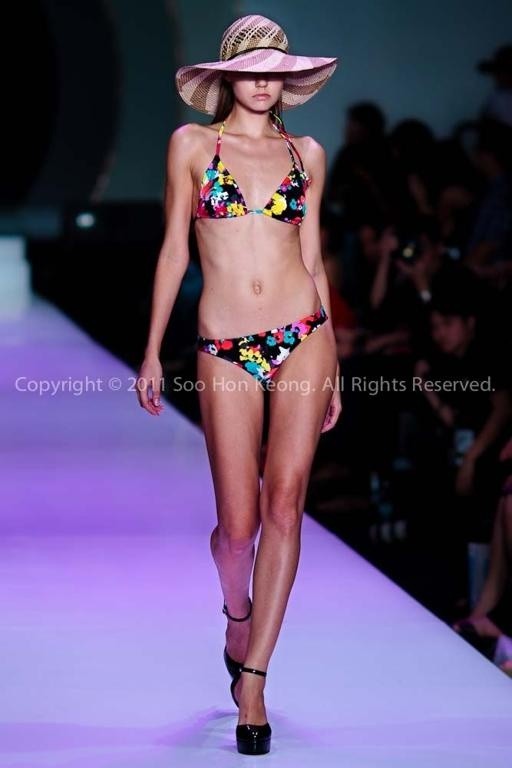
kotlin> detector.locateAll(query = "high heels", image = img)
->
[222,597,271,755]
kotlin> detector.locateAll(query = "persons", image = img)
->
[302,40,510,677]
[132,14,345,757]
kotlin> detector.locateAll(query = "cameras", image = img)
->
[397,239,419,262]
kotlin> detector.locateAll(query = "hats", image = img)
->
[175,14,339,117]
